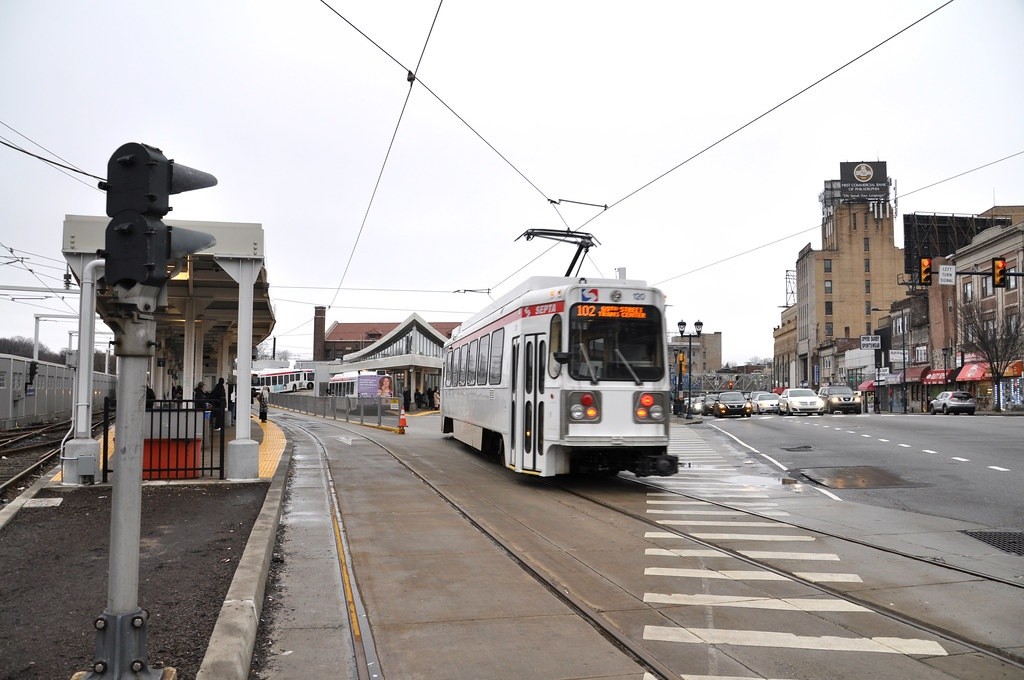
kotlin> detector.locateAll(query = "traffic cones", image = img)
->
[395,405,409,427]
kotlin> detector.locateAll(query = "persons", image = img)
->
[172,383,183,403]
[427,386,440,410]
[251,388,258,418]
[377,376,392,397]
[258,385,269,423]
[414,389,428,410]
[227,378,236,421]
[403,387,410,412]
[146,386,155,409]
[210,378,226,431]
[194,382,206,409]
[874,397,880,412]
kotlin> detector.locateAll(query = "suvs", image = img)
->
[818,386,828,398]
[929,390,977,415]
[819,387,862,415]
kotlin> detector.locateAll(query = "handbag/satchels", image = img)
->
[257,394,262,402]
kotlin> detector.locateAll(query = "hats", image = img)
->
[198,381,206,387]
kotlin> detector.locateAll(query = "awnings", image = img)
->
[858,380,875,392]
[922,369,954,384]
[985,360,1024,377]
[884,372,904,384]
[948,362,991,383]
[905,366,931,382]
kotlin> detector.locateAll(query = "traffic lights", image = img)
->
[96,143,218,287]
[992,257,1006,288]
[919,256,932,286]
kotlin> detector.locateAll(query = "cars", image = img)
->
[713,391,752,418]
[752,393,780,415]
[742,390,769,402]
[684,394,720,416]
[778,388,825,416]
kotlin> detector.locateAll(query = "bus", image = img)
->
[326,369,393,415]
[251,368,315,393]
[440,228,679,486]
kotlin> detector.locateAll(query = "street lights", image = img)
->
[672,347,687,417]
[677,319,704,420]
[942,347,949,391]
[871,307,908,414]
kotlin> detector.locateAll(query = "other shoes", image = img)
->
[262,420,267,423]
[214,427,221,431]
